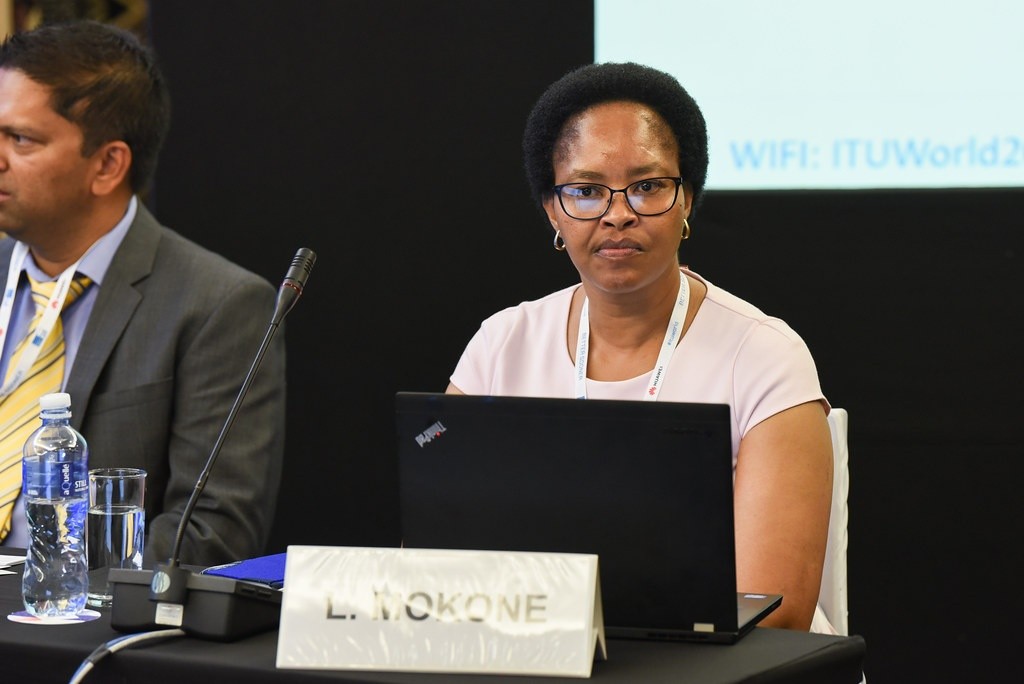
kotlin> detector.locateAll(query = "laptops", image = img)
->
[393,392,784,645]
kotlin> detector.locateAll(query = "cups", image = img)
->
[85,468,147,608]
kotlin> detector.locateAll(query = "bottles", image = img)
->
[21,392,89,619]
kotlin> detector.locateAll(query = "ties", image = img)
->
[0,273,93,543]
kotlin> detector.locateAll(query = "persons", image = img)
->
[442,63,833,633]
[0,21,288,564]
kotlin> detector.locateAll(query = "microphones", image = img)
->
[110,247,318,641]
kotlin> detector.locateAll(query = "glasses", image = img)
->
[553,177,683,220]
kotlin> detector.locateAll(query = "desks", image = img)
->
[0,546,867,684]
